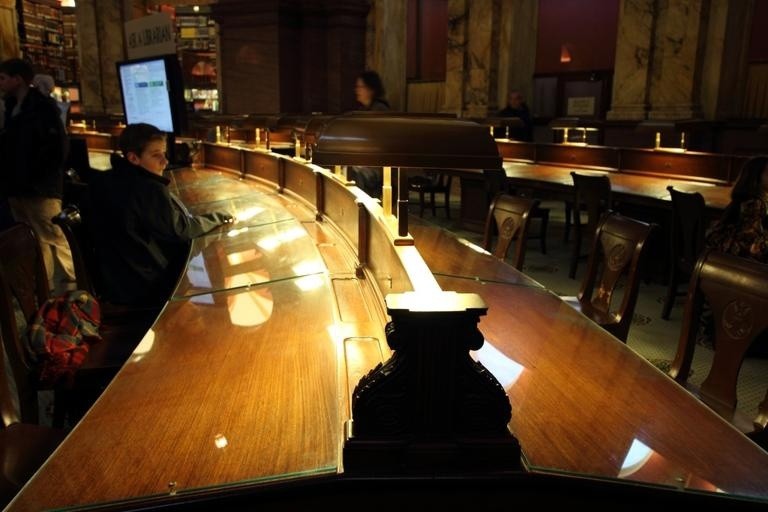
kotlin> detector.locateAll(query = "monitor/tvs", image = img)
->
[116,54,188,171]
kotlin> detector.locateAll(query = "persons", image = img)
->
[33,74,55,98]
[83,123,237,307]
[496,90,534,143]
[350,71,393,191]
[0,58,78,292]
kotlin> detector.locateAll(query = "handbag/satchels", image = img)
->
[19,287,102,384]
